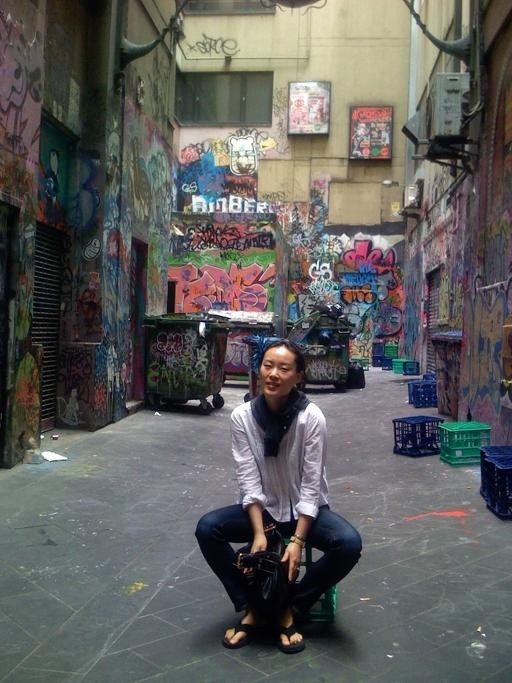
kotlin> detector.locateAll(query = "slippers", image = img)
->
[221,619,255,650]
[270,619,306,654]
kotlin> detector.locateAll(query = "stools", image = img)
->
[279,537,338,624]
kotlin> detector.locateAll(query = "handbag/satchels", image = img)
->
[234,550,292,606]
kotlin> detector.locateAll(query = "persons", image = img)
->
[193,337,363,653]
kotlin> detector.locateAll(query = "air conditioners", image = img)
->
[425,72,472,142]
[402,185,419,209]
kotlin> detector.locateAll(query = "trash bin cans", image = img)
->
[145,312,235,415]
[207,307,274,385]
[287,319,349,388]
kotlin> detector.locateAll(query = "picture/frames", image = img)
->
[288,82,331,135]
[348,106,393,161]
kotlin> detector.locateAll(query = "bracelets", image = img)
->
[293,534,305,542]
[289,536,305,547]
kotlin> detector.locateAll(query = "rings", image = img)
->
[295,567,301,571]
